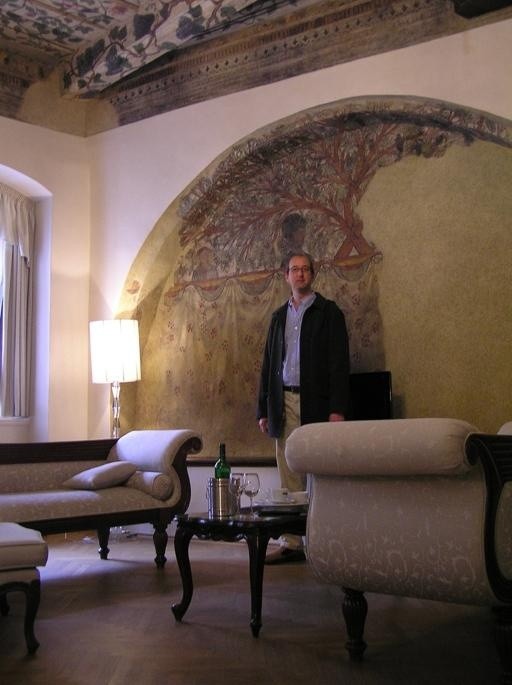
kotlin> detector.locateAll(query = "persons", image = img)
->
[255,252,348,565]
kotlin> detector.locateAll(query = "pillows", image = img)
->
[125,472,174,501]
[67,462,137,490]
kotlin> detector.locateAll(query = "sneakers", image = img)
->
[265,547,306,565]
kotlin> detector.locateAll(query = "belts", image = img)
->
[283,386,300,393]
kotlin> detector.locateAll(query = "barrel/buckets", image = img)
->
[207,477,242,518]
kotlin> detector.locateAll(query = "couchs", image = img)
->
[1,428,205,571]
[282,417,511,664]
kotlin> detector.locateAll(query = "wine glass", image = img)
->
[242,474,261,519]
[227,472,248,520]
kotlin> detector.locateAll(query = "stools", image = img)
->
[1,520,50,656]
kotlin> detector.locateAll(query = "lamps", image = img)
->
[81,319,144,547]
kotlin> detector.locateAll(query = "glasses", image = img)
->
[290,265,310,272]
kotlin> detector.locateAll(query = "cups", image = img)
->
[291,491,308,504]
[273,487,289,502]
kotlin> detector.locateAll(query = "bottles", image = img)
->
[214,442,231,479]
